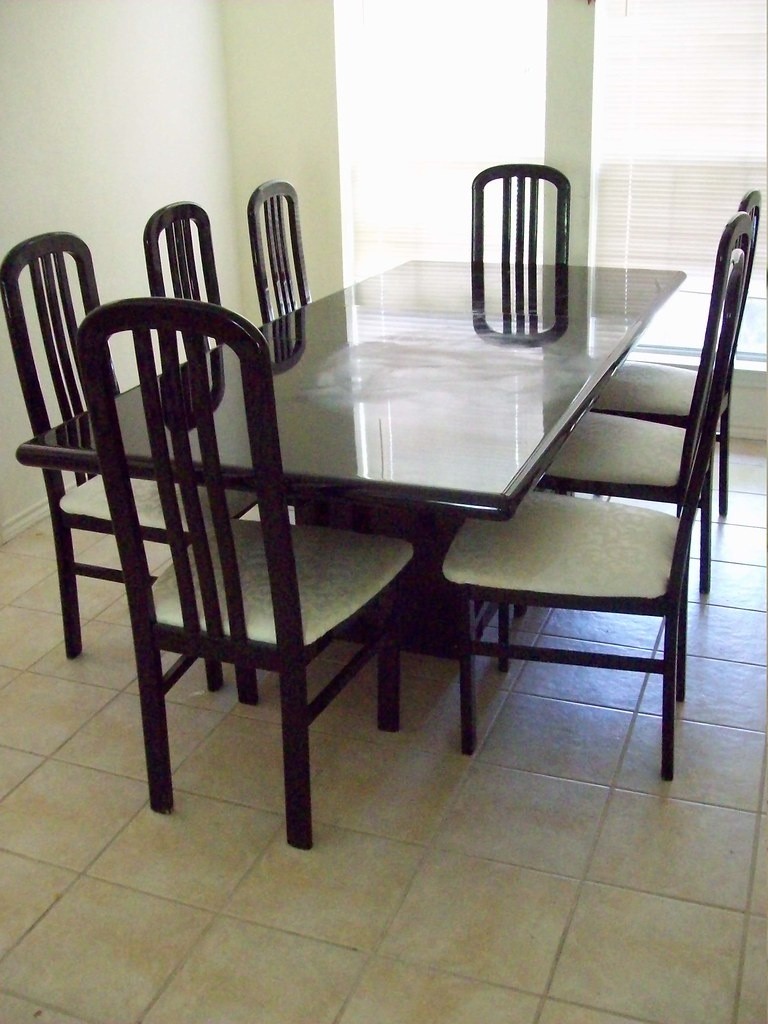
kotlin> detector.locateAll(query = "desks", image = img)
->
[15,259,687,665]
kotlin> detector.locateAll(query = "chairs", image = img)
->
[0,163,762,853]
